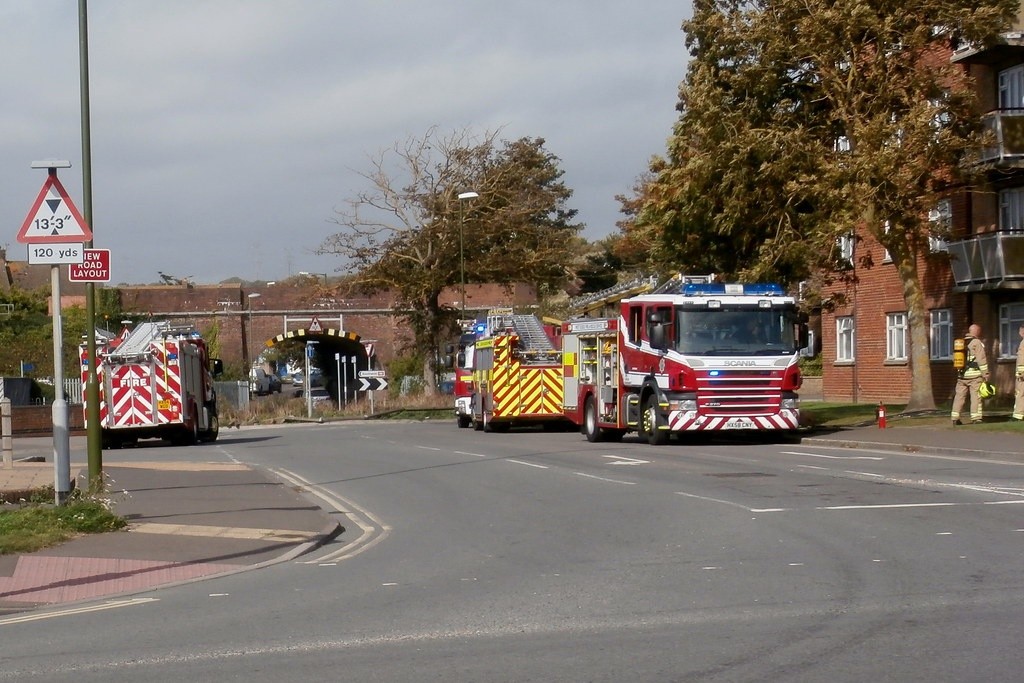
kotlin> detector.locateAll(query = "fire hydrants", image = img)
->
[875,400,885,429]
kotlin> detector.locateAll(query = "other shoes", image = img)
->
[953,420,962,424]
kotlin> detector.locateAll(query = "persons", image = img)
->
[950,323,989,425]
[740,313,767,346]
[1005,323,1024,421]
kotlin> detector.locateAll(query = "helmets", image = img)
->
[979,382,996,399]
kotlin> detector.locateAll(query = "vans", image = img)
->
[249,366,271,396]
[268,374,281,393]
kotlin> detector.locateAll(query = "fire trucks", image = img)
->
[559,272,808,444]
[78,319,224,449]
[445,304,563,432]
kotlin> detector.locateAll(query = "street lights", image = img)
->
[460,191,480,339]
[32,159,75,506]
[307,340,319,419]
[248,293,261,404]
[299,272,327,286]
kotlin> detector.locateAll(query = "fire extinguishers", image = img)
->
[876,401,886,429]
